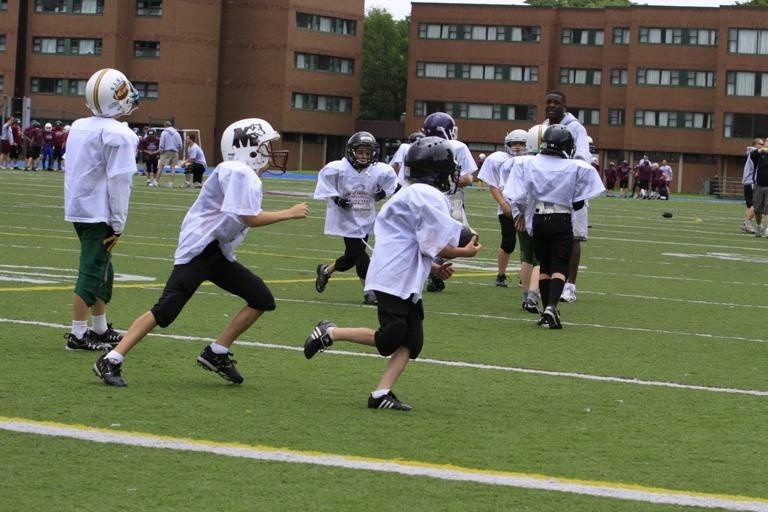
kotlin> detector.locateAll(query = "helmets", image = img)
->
[220,118,289,177]
[84,68,140,118]
[147,130,156,136]
[405,136,461,195]
[424,112,459,138]
[525,124,548,154]
[345,132,379,171]
[541,124,577,159]
[12,117,62,132]
[504,129,528,156]
[408,133,423,142]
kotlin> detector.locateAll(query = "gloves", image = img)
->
[334,196,353,209]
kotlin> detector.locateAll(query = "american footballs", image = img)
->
[662,211,673,217]
[460,225,478,247]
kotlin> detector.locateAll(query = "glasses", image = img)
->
[758,143,764,146]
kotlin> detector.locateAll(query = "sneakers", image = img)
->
[740,222,755,235]
[362,290,378,305]
[764,230,768,238]
[428,274,446,292]
[496,274,513,288]
[89,322,123,345]
[196,345,243,385]
[303,319,338,359]
[518,273,576,328]
[367,389,412,411]
[145,177,205,188]
[92,353,128,387]
[315,263,331,293]
[755,231,762,237]
[62,328,113,352]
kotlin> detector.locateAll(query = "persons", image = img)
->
[313,131,398,306]
[0,117,207,188]
[391,111,480,291]
[745,138,768,239]
[304,137,482,411]
[93,118,310,388]
[61,67,139,353]
[479,93,606,328]
[741,138,768,234]
[605,153,672,200]
